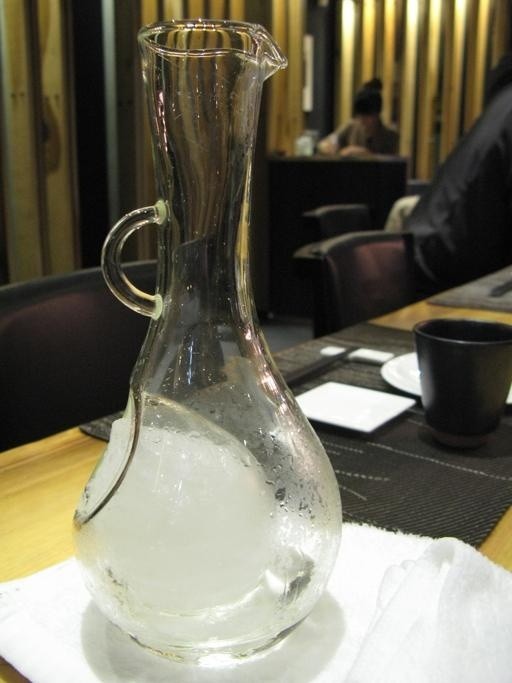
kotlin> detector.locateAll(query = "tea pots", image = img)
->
[71,18,350,670]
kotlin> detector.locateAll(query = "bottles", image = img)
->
[293,136,312,156]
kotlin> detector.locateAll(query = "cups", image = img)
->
[302,129,320,153]
[414,317,511,436]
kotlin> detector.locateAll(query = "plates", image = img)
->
[378,349,512,403]
[289,379,419,439]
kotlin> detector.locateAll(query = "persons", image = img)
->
[399,56,512,295]
[313,77,398,157]
